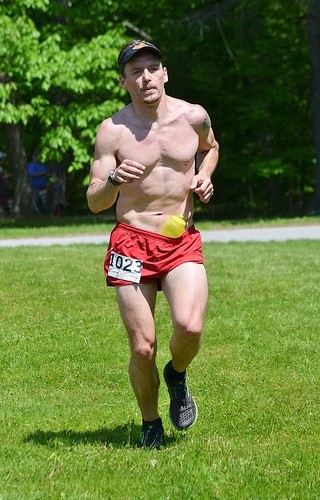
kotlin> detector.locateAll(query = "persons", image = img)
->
[86,39,220,450]
[27,154,53,215]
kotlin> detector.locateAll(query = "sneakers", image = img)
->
[163,360,198,431]
[140,417,164,451]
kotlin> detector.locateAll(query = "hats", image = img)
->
[118,39,162,77]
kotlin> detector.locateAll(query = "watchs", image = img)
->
[108,168,122,186]
